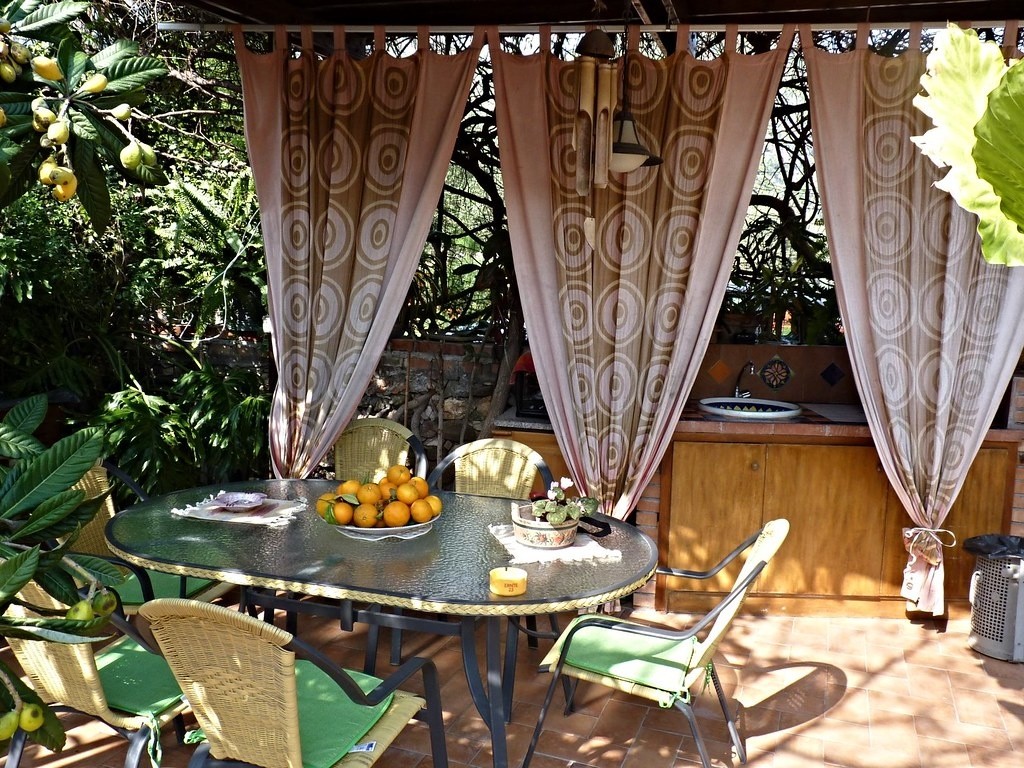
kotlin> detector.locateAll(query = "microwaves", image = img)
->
[516,370,549,420]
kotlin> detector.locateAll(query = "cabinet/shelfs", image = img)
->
[665,437,1010,601]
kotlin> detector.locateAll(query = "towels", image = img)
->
[508,351,535,385]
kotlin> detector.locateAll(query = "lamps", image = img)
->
[610,26,664,174]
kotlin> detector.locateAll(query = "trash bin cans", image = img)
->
[961,534,1024,663]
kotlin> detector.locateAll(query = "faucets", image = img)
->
[735,360,754,398]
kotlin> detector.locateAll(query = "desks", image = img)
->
[104,478,659,768]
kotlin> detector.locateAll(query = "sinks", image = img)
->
[698,397,802,422]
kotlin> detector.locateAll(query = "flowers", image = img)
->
[532,477,599,525]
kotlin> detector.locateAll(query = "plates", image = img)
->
[211,492,267,512]
[320,511,442,535]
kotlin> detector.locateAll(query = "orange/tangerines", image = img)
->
[316,465,442,527]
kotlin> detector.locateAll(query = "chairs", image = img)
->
[0,418,792,768]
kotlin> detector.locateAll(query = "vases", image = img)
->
[511,502,579,549]
